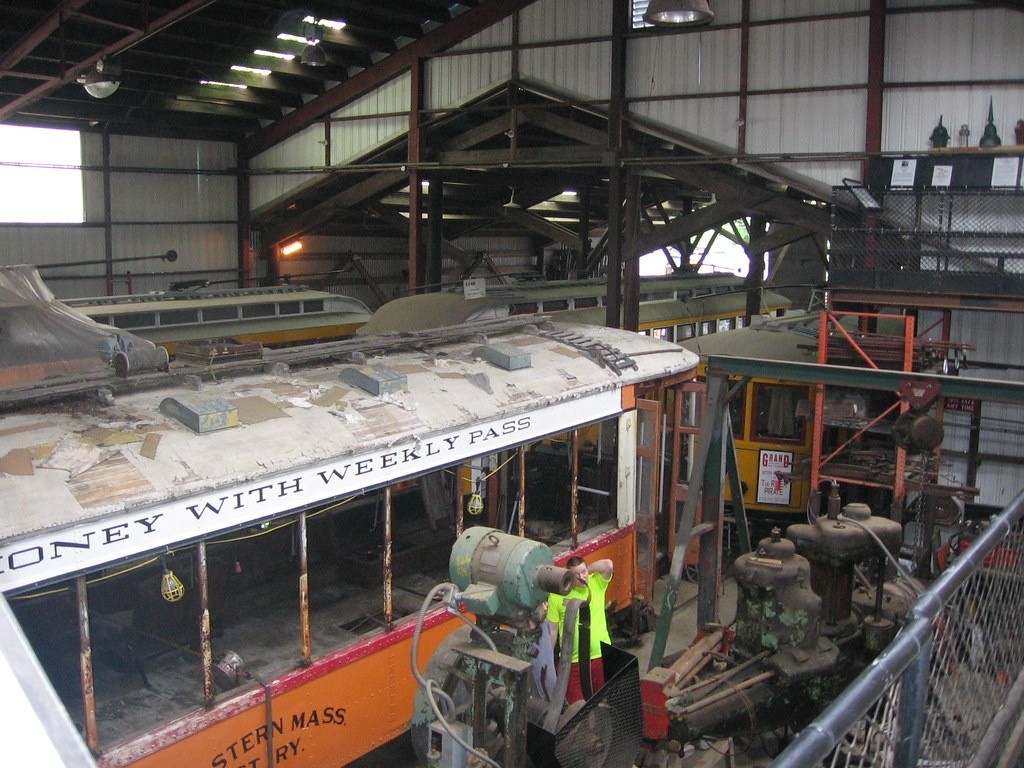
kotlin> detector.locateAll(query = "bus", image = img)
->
[0,311,700,768]
[0,285,373,391]
[665,308,913,518]
[354,266,792,452]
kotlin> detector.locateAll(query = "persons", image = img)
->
[546,557,613,703]
[510,599,556,703]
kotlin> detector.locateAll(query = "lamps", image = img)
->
[83,68,121,98]
[301,16,326,67]
[503,185,521,209]
[960,124,970,147]
[96,55,124,75]
[929,114,950,147]
[642,0,715,28]
[159,553,185,602]
[467,477,484,515]
